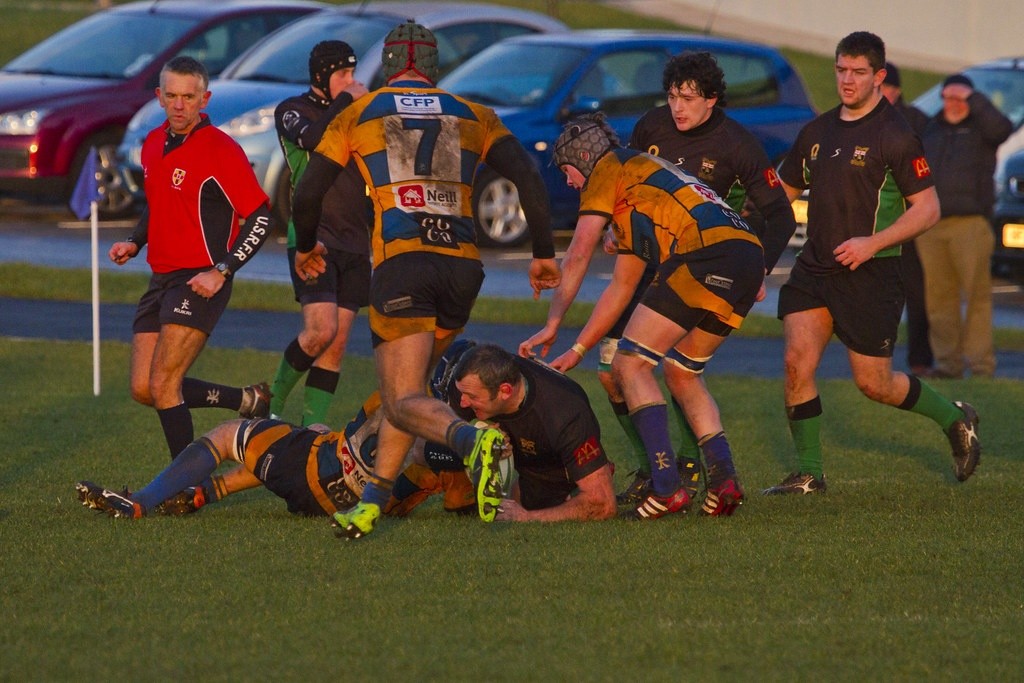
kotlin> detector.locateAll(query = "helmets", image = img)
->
[553,120,611,178]
[311,40,357,88]
[382,19,439,86]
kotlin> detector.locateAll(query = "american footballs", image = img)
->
[463,418,513,496]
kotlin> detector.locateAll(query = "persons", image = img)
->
[75,390,513,519]
[518,112,766,520]
[883,62,934,379]
[597,53,796,510]
[292,19,561,541]
[915,74,1013,377]
[268,41,373,430]
[755,31,979,497]
[109,56,272,463]
[413,340,617,522]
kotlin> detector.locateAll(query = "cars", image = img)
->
[0,0,352,220]
[437,29,820,249]
[785,55,1024,275]
[112,1,607,248]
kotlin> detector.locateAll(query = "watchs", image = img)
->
[214,263,232,280]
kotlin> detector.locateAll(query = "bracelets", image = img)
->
[571,342,588,359]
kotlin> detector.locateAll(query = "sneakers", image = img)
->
[464,428,509,524]
[761,472,826,494]
[331,501,381,541]
[696,479,744,516]
[240,382,274,418]
[156,484,209,515]
[620,486,693,520]
[616,471,653,504]
[942,402,984,482]
[77,480,148,518]
[676,454,708,499]
